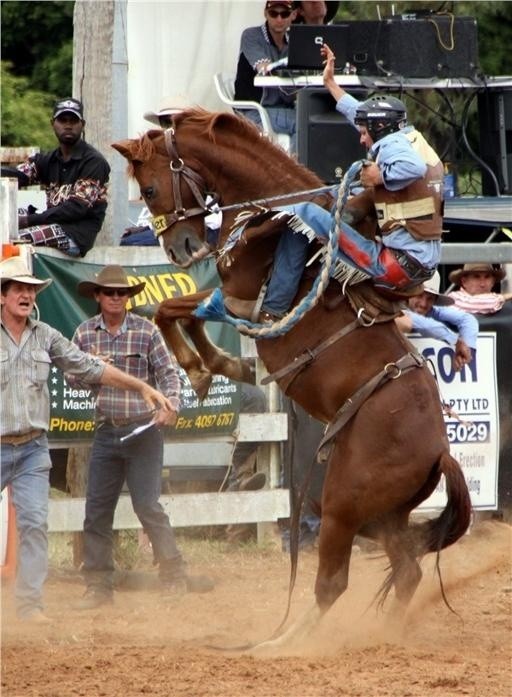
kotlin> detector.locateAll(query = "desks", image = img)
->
[253,73,512,194]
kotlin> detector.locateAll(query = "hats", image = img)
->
[266,1,291,9]
[53,99,83,120]
[422,271,454,306]
[449,264,506,285]
[78,265,146,298]
[292,1,339,23]
[1,256,53,295]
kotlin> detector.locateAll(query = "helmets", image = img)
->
[354,96,407,143]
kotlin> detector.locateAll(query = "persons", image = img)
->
[217,378,269,494]
[223,39,446,326]
[0,249,179,629]
[233,0,306,156]
[290,2,332,27]
[395,265,477,367]
[68,264,187,609]
[7,98,111,257]
[447,262,512,318]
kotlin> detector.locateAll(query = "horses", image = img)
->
[111,104,471,656]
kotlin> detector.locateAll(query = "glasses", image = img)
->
[268,9,291,19]
[99,288,131,297]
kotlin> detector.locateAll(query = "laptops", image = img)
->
[274,24,349,70]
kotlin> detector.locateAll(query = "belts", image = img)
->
[1,431,42,445]
[107,415,154,426]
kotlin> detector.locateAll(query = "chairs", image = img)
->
[214,72,291,160]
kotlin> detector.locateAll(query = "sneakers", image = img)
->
[71,584,114,608]
[160,577,185,603]
[224,295,277,327]
[27,611,52,625]
[238,472,266,489]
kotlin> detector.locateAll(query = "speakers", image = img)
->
[420,16,480,78]
[478,91,512,196]
[334,21,437,79]
